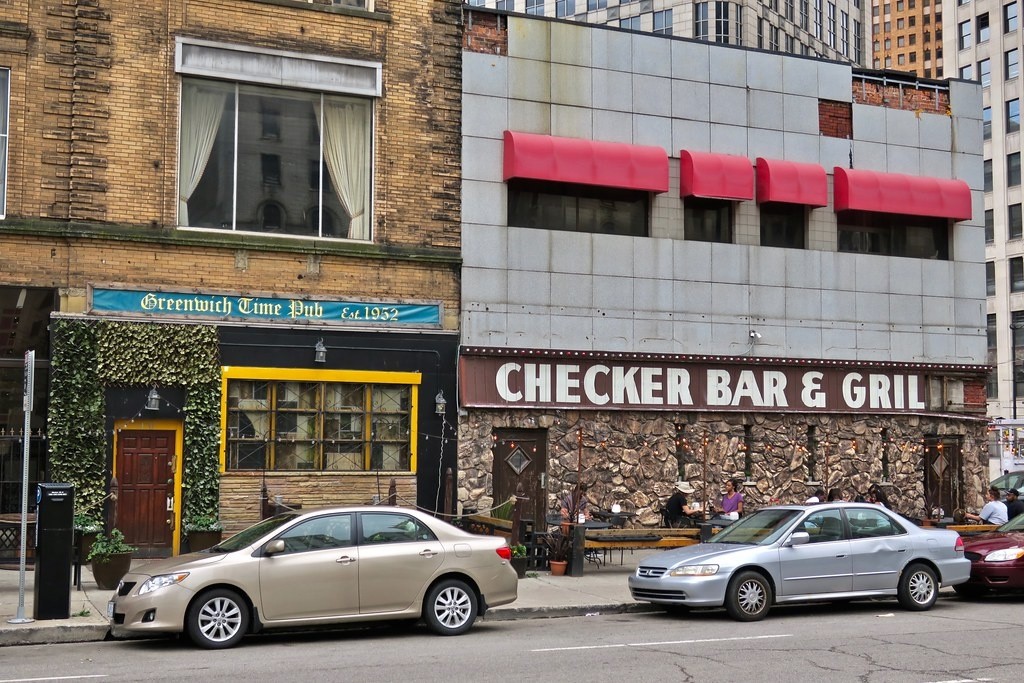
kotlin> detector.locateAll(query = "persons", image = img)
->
[561,486,590,536]
[666,478,1024,536]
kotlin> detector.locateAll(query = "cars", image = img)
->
[989,470,1024,505]
[950,511,1024,599]
[105,502,520,649]
[626,501,972,623]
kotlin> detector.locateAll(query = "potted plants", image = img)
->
[916,487,945,526]
[510,545,528,578]
[89,528,140,590]
[542,533,572,575]
[558,487,589,534]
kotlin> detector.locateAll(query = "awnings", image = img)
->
[503,128,973,223]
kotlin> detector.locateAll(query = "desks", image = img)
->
[590,512,635,528]
[934,516,975,525]
[547,518,614,568]
[692,510,720,528]
[0,513,82,590]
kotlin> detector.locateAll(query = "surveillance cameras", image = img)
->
[757,333,762,339]
[750,333,755,338]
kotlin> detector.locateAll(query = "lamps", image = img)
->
[314,339,327,363]
[144,387,159,411]
[750,331,763,339]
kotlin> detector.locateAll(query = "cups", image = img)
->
[372,494,381,506]
[274,496,283,505]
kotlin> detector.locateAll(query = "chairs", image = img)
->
[308,204,339,238]
[256,201,289,235]
[659,508,688,552]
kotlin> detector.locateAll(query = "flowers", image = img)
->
[184,516,225,539]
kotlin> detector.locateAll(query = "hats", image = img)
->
[675,482,696,493]
[1004,488,1020,497]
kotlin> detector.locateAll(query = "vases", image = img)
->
[189,530,222,552]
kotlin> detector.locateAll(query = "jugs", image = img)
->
[692,502,700,510]
[611,503,621,514]
[578,513,585,524]
[729,512,739,520]
